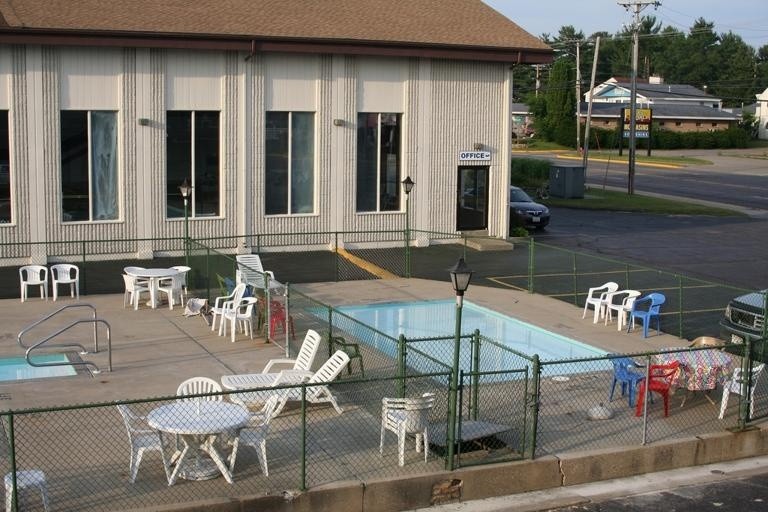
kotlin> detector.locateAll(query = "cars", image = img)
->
[462,185,552,230]
[718,286,767,359]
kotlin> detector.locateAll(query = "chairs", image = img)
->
[605,350,679,416]
[379,392,439,464]
[207,254,297,343]
[221,329,365,415]
[689,335,726,352]
[716,362,766,420]
[115,375,280,484]
[119,263,191,310]
[18,262,81,302]
[581,279,666,339]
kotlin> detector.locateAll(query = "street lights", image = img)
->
[177,180,195,292]
[400,175,416,278]
[445,253,474,471]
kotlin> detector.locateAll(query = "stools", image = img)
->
[4,469,54,512]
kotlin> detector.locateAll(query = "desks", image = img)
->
[653,346,732,407]
